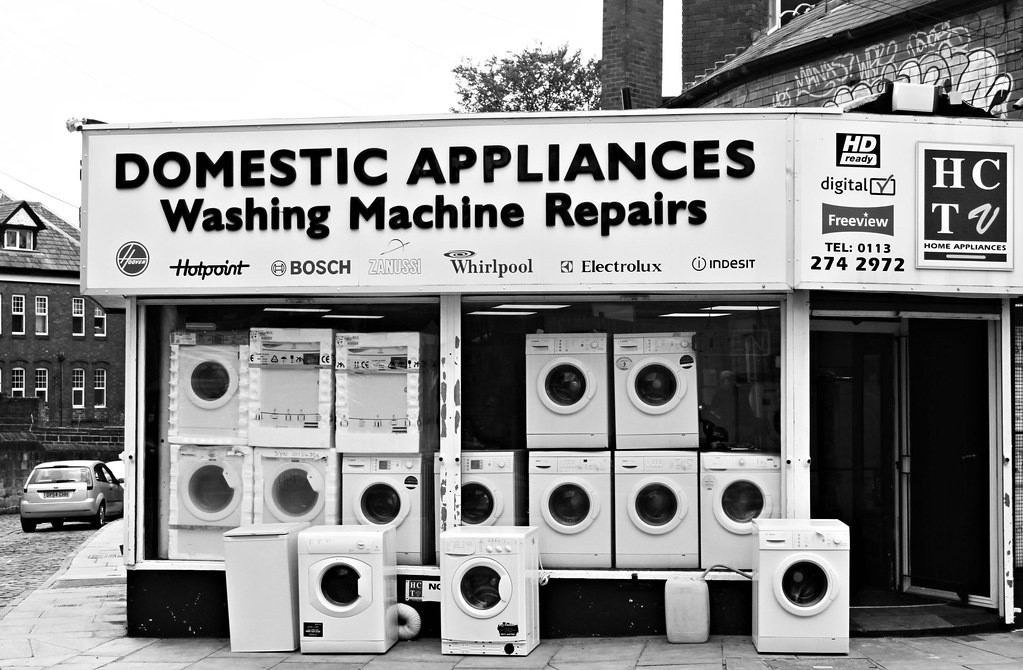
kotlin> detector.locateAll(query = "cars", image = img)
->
[18,459,124,526]
[99,459,124,487]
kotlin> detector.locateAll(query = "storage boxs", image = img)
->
[663,575,710,644]
